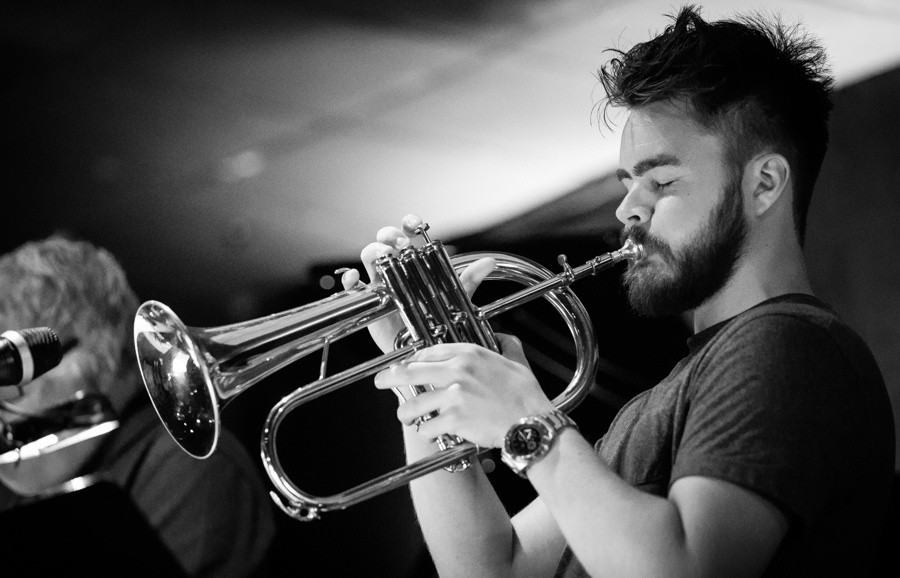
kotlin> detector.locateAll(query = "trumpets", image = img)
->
[135,221,647,523]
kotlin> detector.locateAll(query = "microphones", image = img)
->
[0,327,62,387]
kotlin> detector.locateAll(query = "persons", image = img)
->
[0,233,276,578]
[339,4,900,578]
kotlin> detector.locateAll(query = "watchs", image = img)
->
[501,407,580,478]
